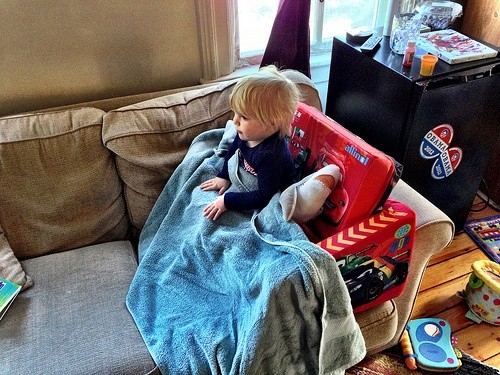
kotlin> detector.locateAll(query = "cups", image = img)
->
[420,55,438,76]
[390,13,422,54]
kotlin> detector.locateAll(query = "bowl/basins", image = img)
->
[416,0,462,30]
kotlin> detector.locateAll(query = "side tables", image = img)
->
[325,33,499,235]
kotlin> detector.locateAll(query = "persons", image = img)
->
[199,66,301,221]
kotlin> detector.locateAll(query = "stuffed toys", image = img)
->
[279,163,340,223]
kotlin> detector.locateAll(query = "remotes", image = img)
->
[360,35,383,53]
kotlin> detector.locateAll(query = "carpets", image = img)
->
[344,341,500,375]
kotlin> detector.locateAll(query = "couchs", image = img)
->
[285,100,416,311]
[0,70,457,375]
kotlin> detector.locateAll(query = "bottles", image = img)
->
[403,40,416,66]
